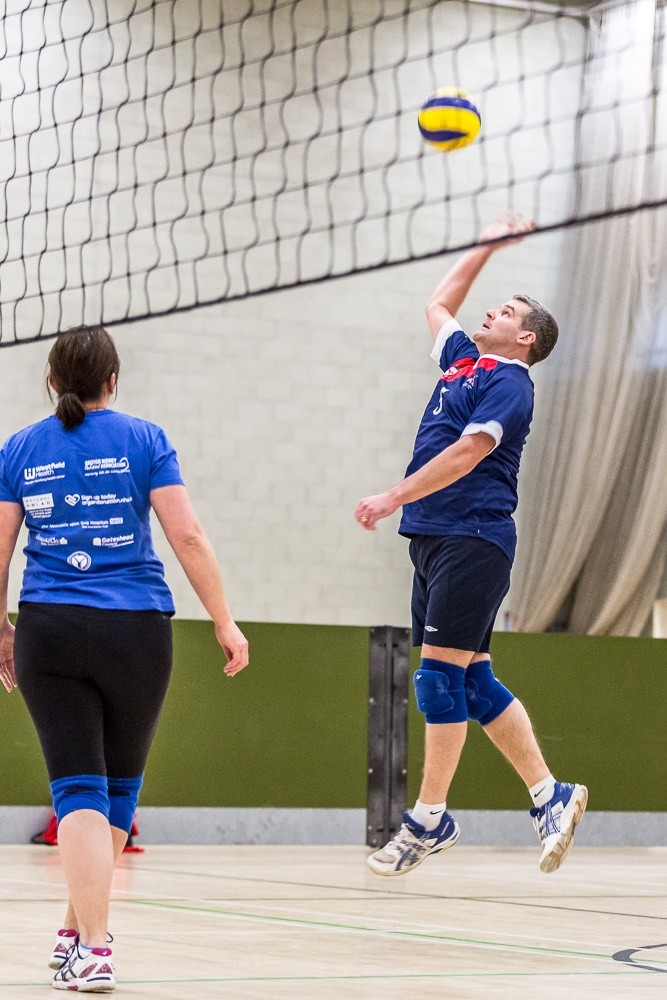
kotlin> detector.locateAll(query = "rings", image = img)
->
[360,516,369,522]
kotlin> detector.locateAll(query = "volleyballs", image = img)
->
[417,87,481,151]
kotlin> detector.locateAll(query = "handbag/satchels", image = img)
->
[30,806,145,852]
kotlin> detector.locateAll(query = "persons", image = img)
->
[0,326,251,992]
[354,214,589,875]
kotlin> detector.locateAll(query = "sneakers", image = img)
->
[52,933,116,992]
[48,928,77,970]
[529,781,588,873]
[367,809,461,876]
